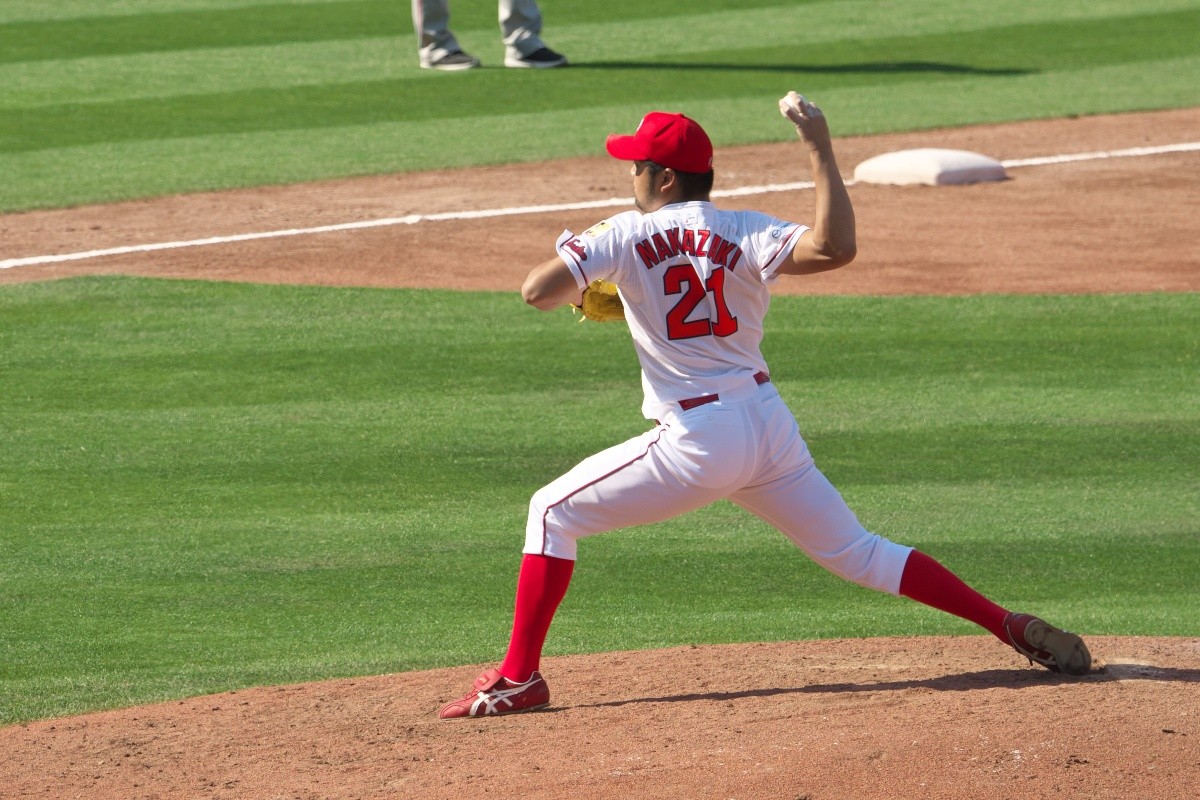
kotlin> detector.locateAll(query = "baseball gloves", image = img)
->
[568,280,626,325]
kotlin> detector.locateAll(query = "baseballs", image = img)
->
[779,93,807,118]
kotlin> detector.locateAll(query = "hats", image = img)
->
[606,111,715,174]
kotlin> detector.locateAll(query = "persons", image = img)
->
[437,91,1092,719]
[411,0,569,70]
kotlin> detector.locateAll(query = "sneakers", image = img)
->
[419,50,480,71]
[1010,612,1091,675]
[438,666,549,716]
[504,45,568,69]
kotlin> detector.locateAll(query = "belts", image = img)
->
[654,371,770,425]
